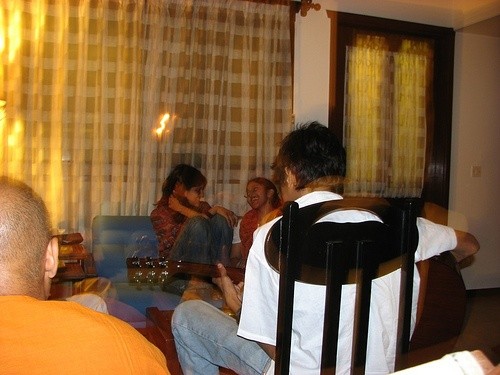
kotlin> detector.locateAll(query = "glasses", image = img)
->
[243,186,261,198]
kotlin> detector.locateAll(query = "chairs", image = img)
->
[274,197,418,375]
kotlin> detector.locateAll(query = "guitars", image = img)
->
[123,245,470,349]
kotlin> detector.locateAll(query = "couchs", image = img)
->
[93,216,159,278]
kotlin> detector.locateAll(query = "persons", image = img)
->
[151,165,239,302]
[0,177,170,375]
[172,121,480,375]
[239,177,278,247]
